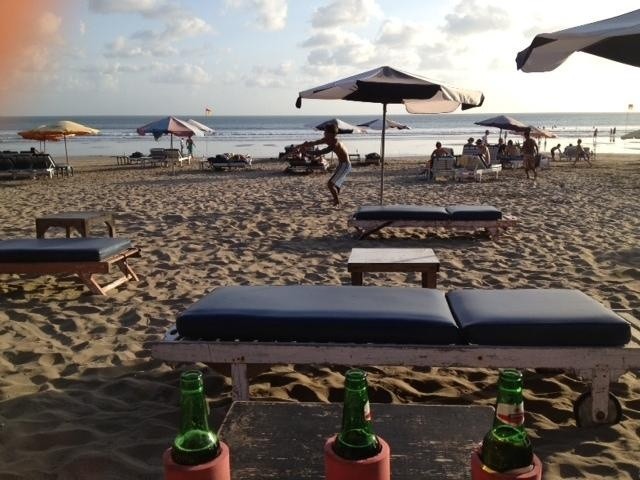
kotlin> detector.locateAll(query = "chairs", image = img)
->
[212,155,252,171]
[0,150,65,181]
[431,144,499,183]
[500,156,549,170]
[364,156,381,166]
[349,154,363,164]
[288,159,330,175]
[559,146,591,162]
[149,148,191,169]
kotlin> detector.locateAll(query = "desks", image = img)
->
[117,155,153,169]
[199,160,209,171]
[346,247,441,287]
[35,210,117,240]
[216,399,495,480]
[56,163,69,180]
[415,167,430,183]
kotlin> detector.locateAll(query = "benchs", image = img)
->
[349,206,520,242]
[0,238,142,296]
[150,284,640,430]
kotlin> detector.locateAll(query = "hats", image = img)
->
[476,139,482,144]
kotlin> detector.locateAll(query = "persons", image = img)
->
[504,138,518,160]
[593,128,598,140]
[299,124,350,207]
[474,139,491,168]
[419,141,449,175]
[466,137,474,146]
[495,137,507,159]
[482,130,489,144]
[211,144,331,172]
[186,136,195,160]
[180,138,184,152]
[573,139,592,168]
[521,132,539,180]
[550,143,561,159]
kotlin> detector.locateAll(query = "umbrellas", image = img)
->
[186,119,219,136]
[474,115,528,140]
[515,9,640,74]
[509,126,556,139]
[138,116,205,149]
[357,117,411,156]
[17,120,100,165]
[313,116,366,165]
[295,65,485,205]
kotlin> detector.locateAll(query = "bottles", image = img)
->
[335,370,380,459]
[174,370,219,463]
[483,370,536,473]
